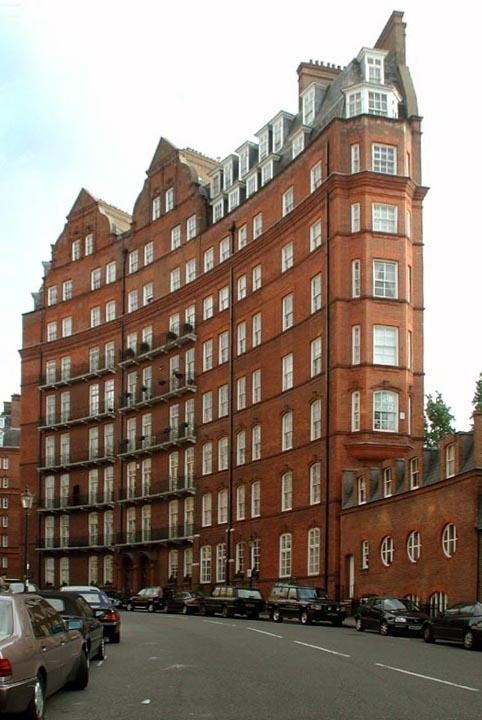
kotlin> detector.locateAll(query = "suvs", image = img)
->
[267,584,347,625]
[204,586,266,619]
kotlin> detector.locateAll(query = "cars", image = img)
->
[423,602,482,650]
[355,596,429,636]
[101,586,201,615]
[0,584,123,713]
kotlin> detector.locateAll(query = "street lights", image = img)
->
[20,488,34,585]
